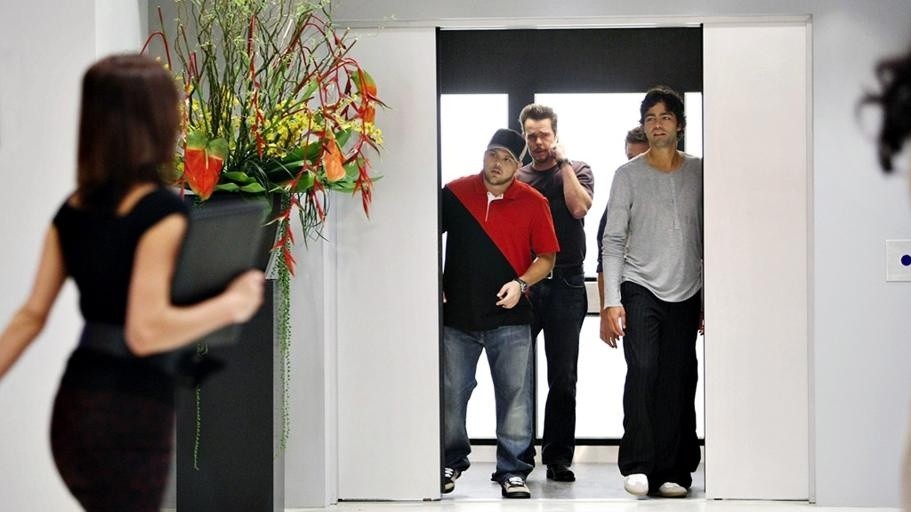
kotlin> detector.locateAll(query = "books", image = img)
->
[169,197,272,346]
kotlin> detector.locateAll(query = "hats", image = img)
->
[486,128,529,164]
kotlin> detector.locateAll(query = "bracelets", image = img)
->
[555,157,572,166]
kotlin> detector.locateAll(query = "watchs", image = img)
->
[514,279,528,293]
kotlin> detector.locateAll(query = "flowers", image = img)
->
[132,0,395,472]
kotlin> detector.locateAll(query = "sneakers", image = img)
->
[624,473,650,498]
[491,463,575,498]
[443,466,461,493]
[656,481,687,497]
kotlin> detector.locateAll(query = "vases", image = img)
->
[181,190,285,281]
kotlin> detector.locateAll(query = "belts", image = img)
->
[543,266,583,281]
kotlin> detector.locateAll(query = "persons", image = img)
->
[439,128,559,497]
[0,53,262,512]
[513,104,593,482]
